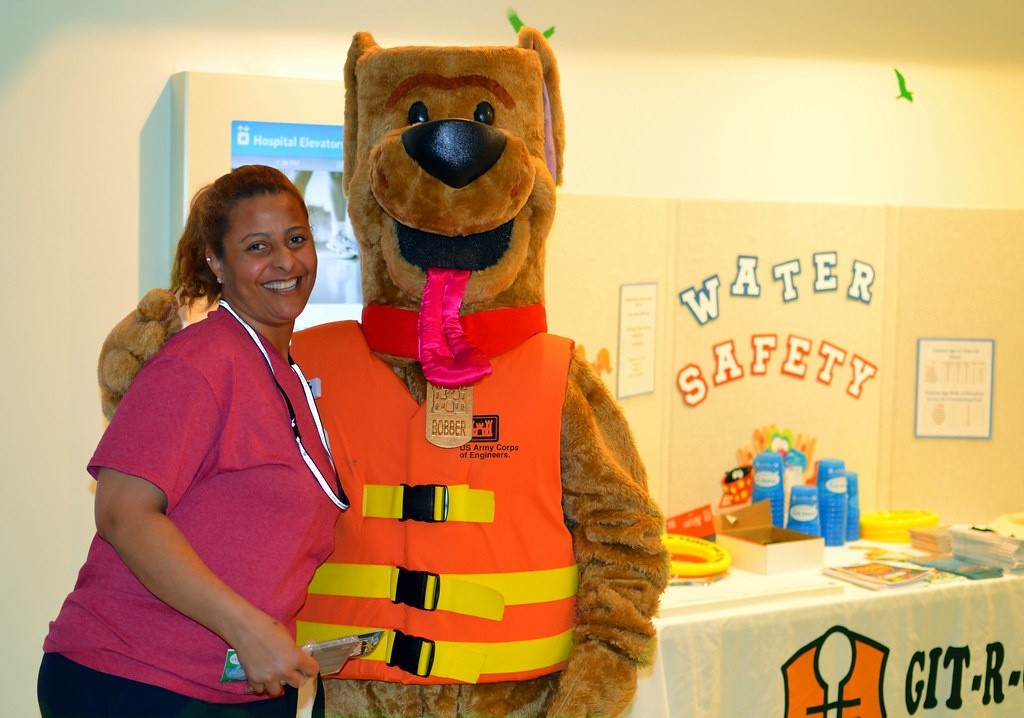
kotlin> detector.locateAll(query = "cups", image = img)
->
[752,453,860,546]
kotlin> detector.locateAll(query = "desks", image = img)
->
[615,523,1024,718]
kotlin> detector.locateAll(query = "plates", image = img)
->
[660,532,730,586]
[860,509,944,545]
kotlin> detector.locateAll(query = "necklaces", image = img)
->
[217,299,350,509]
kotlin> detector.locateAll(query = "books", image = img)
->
[908,524,953,554]
[822,560,934,589]
[911,553,1004,579]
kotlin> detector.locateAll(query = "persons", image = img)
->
[37,164,342,718]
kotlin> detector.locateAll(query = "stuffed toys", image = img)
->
[100,28,670,718]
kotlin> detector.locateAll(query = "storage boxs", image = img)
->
[717,527,825,574]
